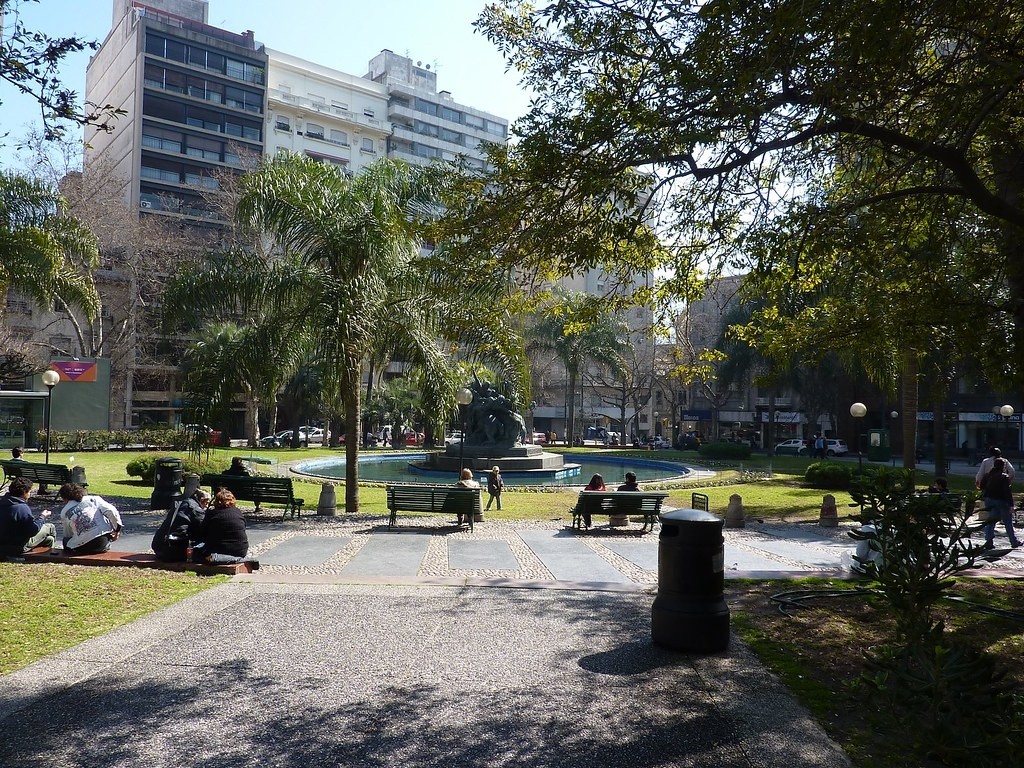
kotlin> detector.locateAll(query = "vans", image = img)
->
[376,424,406,440]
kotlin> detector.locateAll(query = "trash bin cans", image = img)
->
[651,508,732,656]
[150,457,183,509]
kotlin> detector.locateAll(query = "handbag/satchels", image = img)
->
[155,542,188,563]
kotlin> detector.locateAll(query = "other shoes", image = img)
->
[984,540,997,550]
[1011,540,1024,548]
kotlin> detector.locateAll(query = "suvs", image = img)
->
[798,439,848,457]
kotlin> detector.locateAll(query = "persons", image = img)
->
[149,487,250,563]
[485,466,505,511]
[962,440,968,448]
[848,515,891,574]
[0,477,57,553]
[915,449,922,464]
[618,472,645,492]
[584,473,605,527]
[631,429,706,451]
[452,468,480,525]
[221,456,263,512]
[927,478,950,494]
[973,446,1024,549]
[807,434,830,460]
[457,370,529,447]
[383,429,389,447]
[545,430,557,444]
[574,436,585,445]
[59,483,124,555]
[10,446,52,494]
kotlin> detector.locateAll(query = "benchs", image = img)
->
[899,492,965,521]
[0,460,89,505]
[386,484,481,533]
[205,472,306,522]
[567,492,671,532]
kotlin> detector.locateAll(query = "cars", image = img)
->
[388,432,425,446]
[445,432,466,446]
[338,432,378,446]
[261,425,332,449]
[526,432,546,445]
[775,439,807,453]
[655,435,669,449]
[183,423,231,447]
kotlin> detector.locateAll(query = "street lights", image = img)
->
[850,402,867,468]
[891,411,899,467]
[653,411,659,437]
[999,404,1014,474]
[774,410,780,438]
[41,370,60,466]
[456,388,473,481]
[991,405,1002,447]
